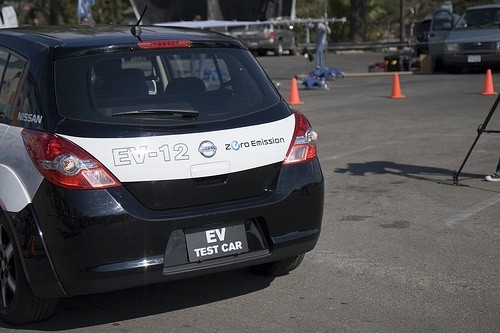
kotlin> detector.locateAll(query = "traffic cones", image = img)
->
[388,73,407,99]
[480,69,499,95]
[287,78,305,105]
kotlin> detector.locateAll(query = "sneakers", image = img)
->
[485,172,500,181]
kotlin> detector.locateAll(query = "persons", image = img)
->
[313,17,331,71]
[91,59,122,92]
[80,16,88,26]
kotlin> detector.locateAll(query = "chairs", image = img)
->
[163,77,206,95]
[92,59,121,86]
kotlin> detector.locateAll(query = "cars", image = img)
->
[241,23,299,57]
[0,24,326,327]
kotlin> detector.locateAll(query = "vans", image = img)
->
[427,4,500,70]
